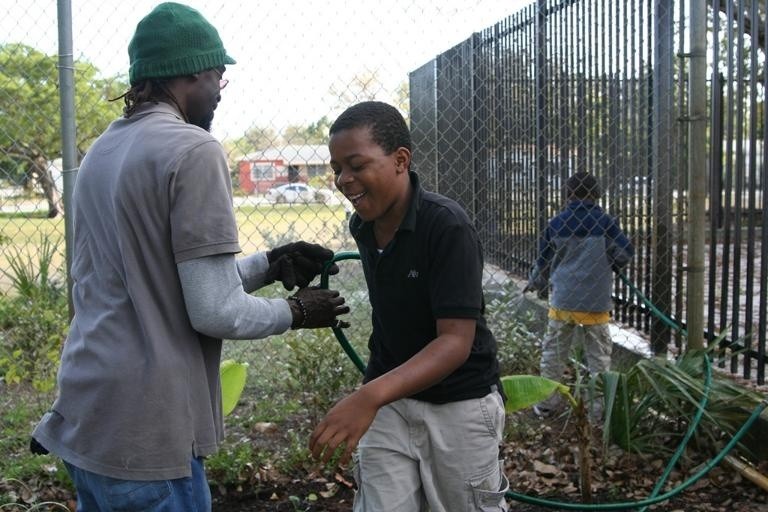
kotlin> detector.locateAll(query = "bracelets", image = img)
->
[286,296,307,330]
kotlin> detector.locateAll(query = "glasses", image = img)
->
[214,68,229,89]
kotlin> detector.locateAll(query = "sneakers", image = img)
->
[534,405,550,417]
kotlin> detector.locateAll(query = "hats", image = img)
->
[128,2,236,84]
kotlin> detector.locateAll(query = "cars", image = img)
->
[266,183,332,203]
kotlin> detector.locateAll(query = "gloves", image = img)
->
[265,241,339,291]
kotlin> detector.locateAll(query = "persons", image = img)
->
[30,2,351,512]
[527,172,635,418]
[309,101,509,512]
